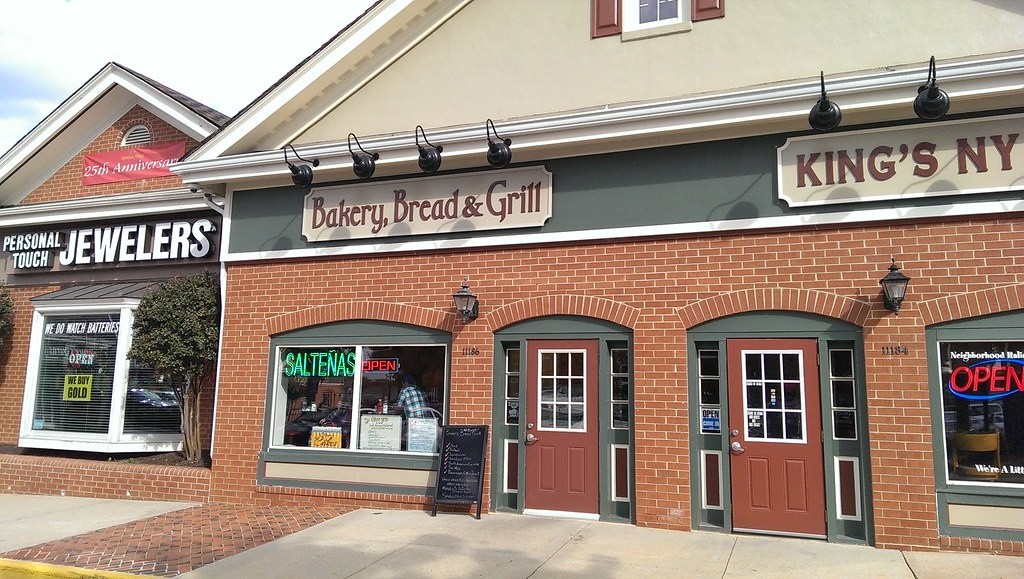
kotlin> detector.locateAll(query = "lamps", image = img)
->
[347,132,379,180]
[808,70,842,132]
[878,257,911,316]
[415,123,443,174]
[485,118,511,170]
[451,275,478,324]
[913,56,950,121]
[282,144,319,188]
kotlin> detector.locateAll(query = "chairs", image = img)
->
[947,431,1001,482]
[408,407,442,449]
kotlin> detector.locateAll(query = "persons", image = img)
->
[395,374,430,419]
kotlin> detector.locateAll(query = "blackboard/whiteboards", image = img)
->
[434,425,491,505]
[359,414,402,450]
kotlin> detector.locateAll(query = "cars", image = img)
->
[945,404,1004,436]
[284,408,443,449]
[131,388,181,431]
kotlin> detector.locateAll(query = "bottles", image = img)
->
[383,395,388,414]
[377,398,383,415]
